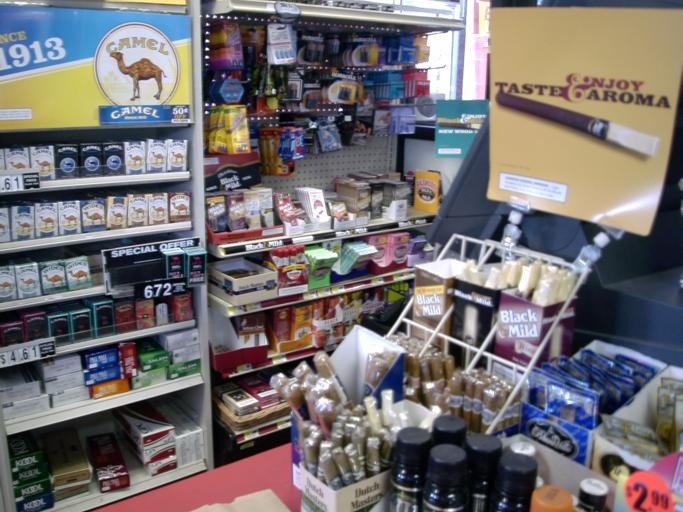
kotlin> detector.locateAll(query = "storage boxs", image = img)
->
[207,258,279,306]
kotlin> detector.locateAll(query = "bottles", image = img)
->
[383,414,610,511]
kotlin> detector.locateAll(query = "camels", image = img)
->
[109,51,166,101]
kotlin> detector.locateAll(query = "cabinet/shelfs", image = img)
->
[201,0,464,465]
[0,0,213,512]
